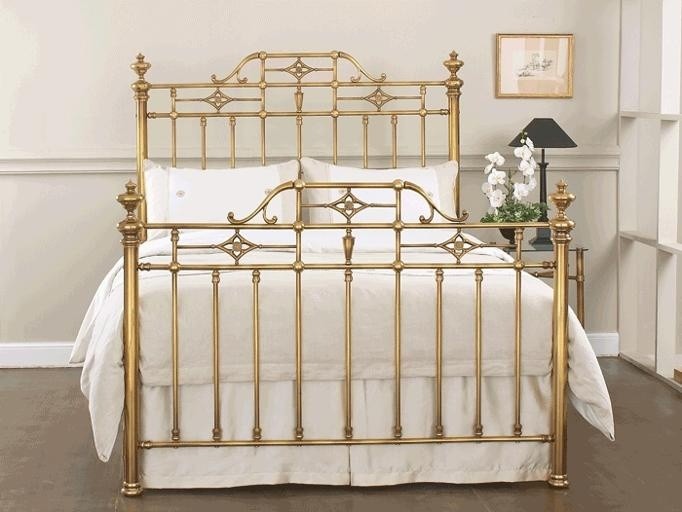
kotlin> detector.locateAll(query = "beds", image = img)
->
[67,52,617,497]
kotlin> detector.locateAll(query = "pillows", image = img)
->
[298,157,460,230]
[143,157,301,239]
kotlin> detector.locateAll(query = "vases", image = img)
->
[497,227,524,252]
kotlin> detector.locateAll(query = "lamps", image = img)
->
[509,117,578,250]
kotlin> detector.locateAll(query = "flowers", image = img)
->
[481,131,549,223]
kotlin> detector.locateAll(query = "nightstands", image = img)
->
[504,241,589,332]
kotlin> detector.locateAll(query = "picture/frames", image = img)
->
[496,32,574,99]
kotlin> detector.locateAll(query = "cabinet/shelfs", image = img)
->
[617,1,682,394]
